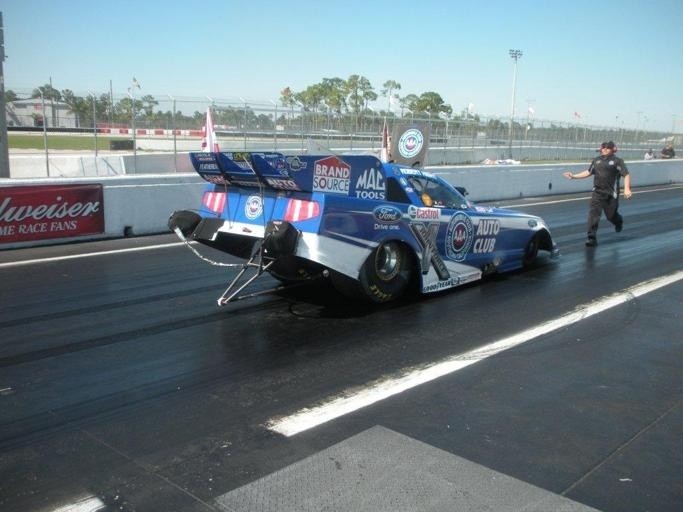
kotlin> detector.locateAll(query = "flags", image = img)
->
[574,112,580,120]
[199,107,218,152]
[380,121,391,163]
[527,106,535,117]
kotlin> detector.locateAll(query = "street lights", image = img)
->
[507,48,522,155]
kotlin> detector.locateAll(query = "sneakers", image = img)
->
[615,216,623,231]
[585,239,597,246]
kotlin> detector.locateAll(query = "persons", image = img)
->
[659,142,677,159]
[643,148,656,160]
[561,141,634,247]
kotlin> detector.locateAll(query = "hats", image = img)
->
[602,141,614,149]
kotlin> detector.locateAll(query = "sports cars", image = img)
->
[482,157,521,166]
[169,137,557,312]
